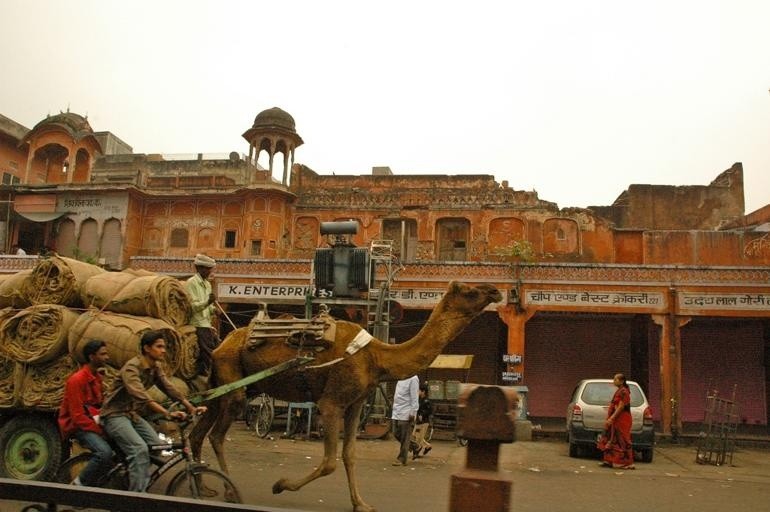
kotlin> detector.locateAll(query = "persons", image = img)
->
[596,373,635,469]
[182,252,224,374]
[57,339,113,488]
[412,382,432,456]
[99,330,208,491]
[389,375,423,467]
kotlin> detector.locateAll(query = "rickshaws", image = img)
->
[423,354,475,447]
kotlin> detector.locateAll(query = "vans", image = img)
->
[565,378,654,464]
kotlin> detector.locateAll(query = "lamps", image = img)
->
[60,160,70,173]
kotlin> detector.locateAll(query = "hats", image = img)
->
[194,254,216,268]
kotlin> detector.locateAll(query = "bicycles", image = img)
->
[244,392,276,438]
[47,406,242,511]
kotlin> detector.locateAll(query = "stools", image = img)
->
[282,400,324,441]
